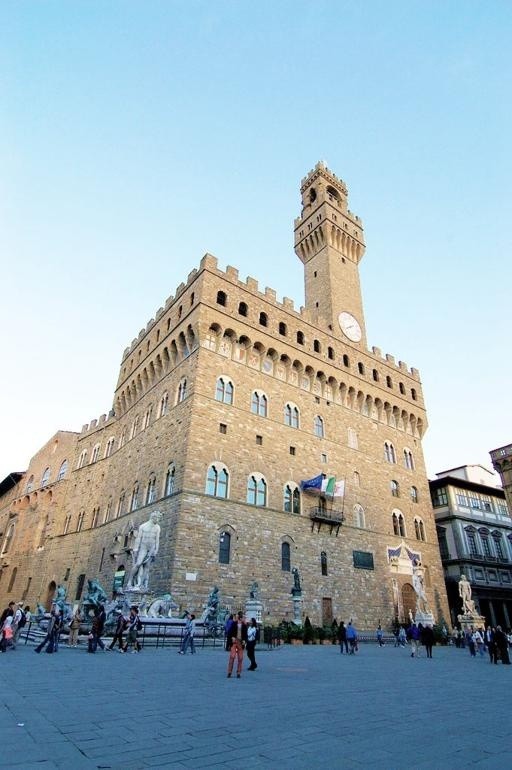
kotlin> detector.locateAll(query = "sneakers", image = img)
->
[227,672,232,678]
[103,643,142,654]
[236,673,240,678]
[177,650,196,655]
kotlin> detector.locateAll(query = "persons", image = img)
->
[56,584,67,603]
[412,569,429,615]
[336,620,347,654]
[183,614,196,655]
[1,601,143,655]
[376,625,386,647]
[390,623,510,664]
[126,509,162,589]
[178,613,195,654]
[84,580,106,604]
[346,622,358,654]
[225,611,259,678]
[291,567,301,590]
[458,574,472,615]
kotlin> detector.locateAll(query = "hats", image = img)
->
[15,601,23,606]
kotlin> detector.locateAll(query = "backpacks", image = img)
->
[137,619,142,631]
[17,609,26,630]
[52,615,61,634]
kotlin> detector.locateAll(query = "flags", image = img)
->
[319,477,335,497]
[301,474,325,494]
[332,480,344,497]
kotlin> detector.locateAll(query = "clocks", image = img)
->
[339,310,364,343]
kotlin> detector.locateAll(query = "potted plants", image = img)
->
[264,620,340,645]
